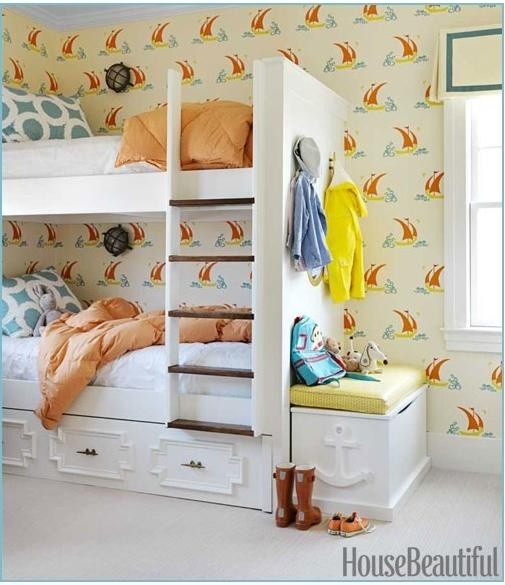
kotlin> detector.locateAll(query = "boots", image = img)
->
[295,464,322,530]
[276,463,297,527]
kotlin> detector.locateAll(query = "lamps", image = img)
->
[101,223,132,259]
[105,60,134,95]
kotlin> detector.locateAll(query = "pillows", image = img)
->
[2,264,84,339]
[0,80,93,145]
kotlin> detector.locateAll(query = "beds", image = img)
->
[0,51,350,515]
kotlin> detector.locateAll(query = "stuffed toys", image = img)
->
[324,335,344,357]
[356,342,388,374]
[31,284,73,337]
[341,350,361,373]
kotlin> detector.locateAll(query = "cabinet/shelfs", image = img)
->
[0,416,248,500]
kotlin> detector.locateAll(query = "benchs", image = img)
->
[289,363,434,523]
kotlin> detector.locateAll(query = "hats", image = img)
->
[294,135,321,178]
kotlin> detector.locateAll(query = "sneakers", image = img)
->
[327,512,345,534]
[339,512,376,537]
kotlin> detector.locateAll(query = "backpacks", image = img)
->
[290,314,347,387]
